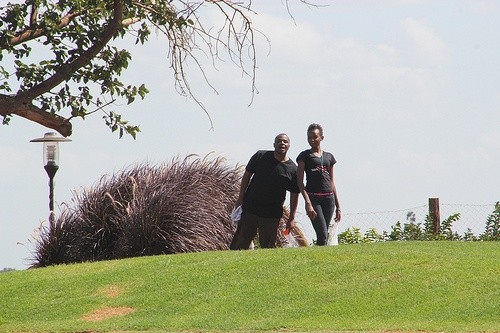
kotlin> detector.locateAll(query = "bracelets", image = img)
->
[336,210,341,212]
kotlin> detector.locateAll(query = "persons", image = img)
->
[230,133,300,250]
[296,124,342,246]
